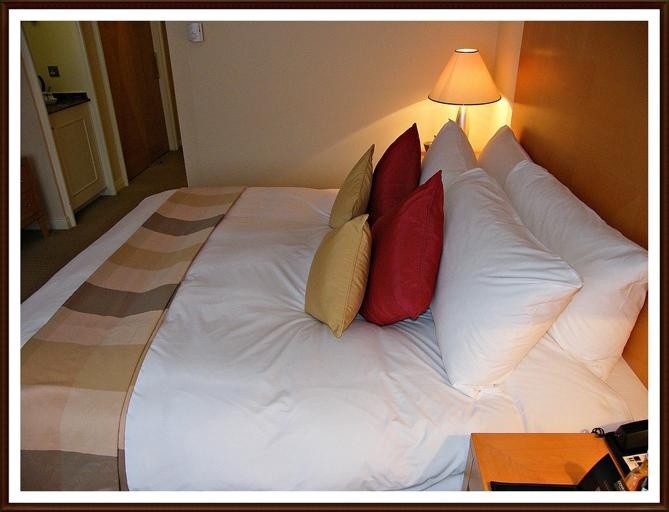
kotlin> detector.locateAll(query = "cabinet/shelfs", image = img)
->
[21,154,52,238]
[41,101,109,219]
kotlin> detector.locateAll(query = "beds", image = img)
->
[20,184,650,490]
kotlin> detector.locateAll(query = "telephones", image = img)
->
[604,420,648,474]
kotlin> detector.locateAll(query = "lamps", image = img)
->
[427,48,502,138]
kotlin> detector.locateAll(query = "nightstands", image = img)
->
[462,430,647,491]
